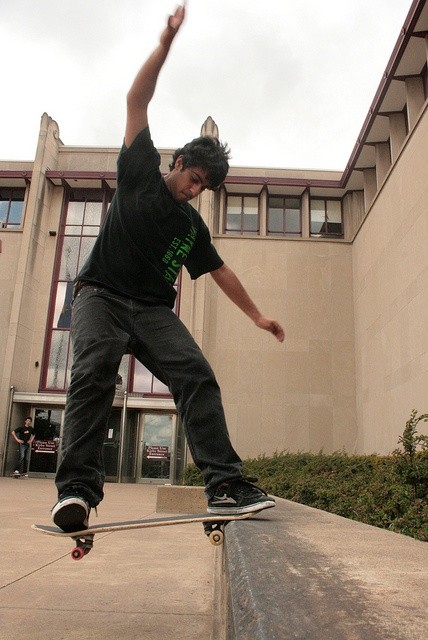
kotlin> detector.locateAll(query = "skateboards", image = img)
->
[31,509,257,560]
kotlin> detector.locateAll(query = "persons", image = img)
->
[11,417,36,478]
[48,2,283,531]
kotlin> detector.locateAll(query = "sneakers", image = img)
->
[50,490,90,532]
[206,477,275,515]
